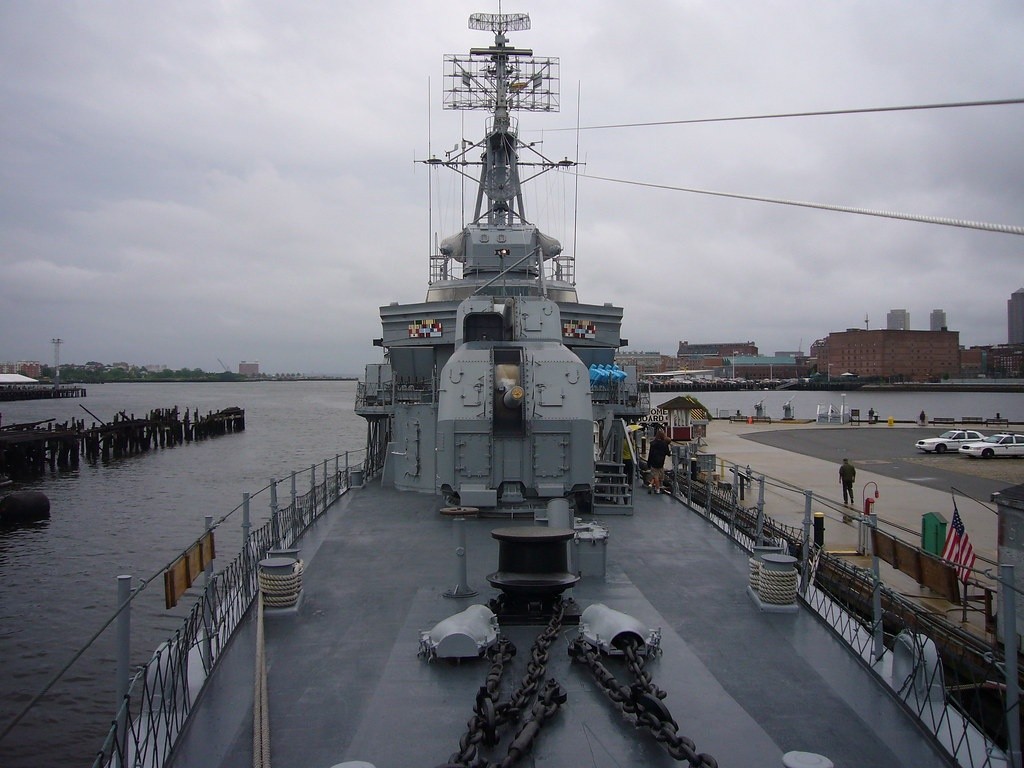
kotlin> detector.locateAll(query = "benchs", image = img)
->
[961,417,983,425]
[751,416,771,424]
[729,416,748,423]
[933,418,955,426]
[986,419,1008,428]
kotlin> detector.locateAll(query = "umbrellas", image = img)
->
[624,425,643,433]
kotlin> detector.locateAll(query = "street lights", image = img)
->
[770,364,773,381]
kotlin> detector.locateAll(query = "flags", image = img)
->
[941,508,976,583]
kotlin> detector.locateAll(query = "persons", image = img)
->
[647,432,671,494]
[920,410,925,424]
[623,433,636,491]
[869,408,874,420]
[839,458,856,504]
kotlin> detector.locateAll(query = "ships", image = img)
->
[2,2,1024,767]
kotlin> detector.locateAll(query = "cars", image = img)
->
[637,377,784,386]
[959,432,1024,460]
[915,428,989,454]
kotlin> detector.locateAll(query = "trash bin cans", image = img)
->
[921,512,949,557]
[888,416,895,426]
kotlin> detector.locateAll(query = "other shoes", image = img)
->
[851,501,854,503]
[844,501,848,504]
[648,484,652,493]
[656,489,660,493]
